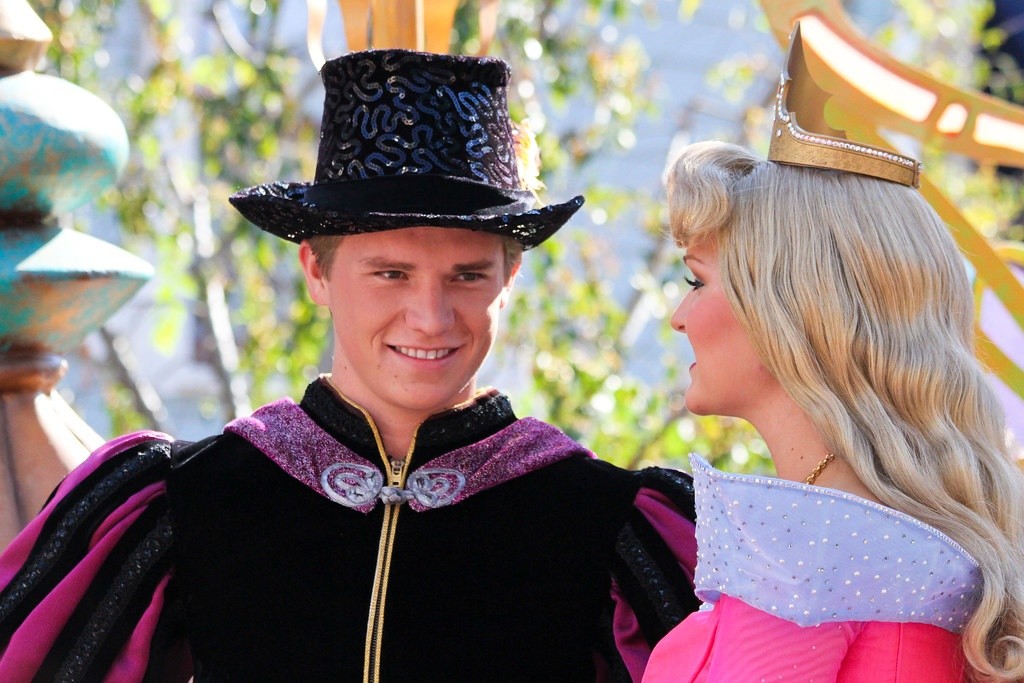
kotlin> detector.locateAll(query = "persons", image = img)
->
[640,66,1024,683]
[0,49,696,683]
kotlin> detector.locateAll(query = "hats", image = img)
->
[228,48,585,251]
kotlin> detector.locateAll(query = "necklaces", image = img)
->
[806,453,835,485]
